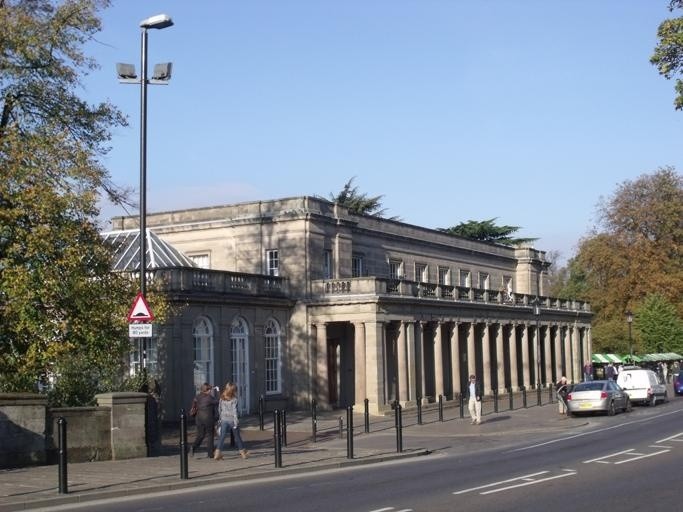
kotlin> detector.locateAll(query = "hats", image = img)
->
[561,376,568,381]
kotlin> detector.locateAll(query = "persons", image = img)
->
[605,359,683,386]
[583,360,593,381]
[465,374,483,425]
[186,382,221,459]
[214,382,251,459]
[555,376,568,413]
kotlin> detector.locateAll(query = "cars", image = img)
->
[673,370,682,397]
[565,369,667,415]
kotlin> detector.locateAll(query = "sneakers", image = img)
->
[208,453,214,459]
[470,419,482,426]
[559,413,567,418]
[189,447,196,458]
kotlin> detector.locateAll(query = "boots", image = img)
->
[214,449,225,461]
[239,448,250,460]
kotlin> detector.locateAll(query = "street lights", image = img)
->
[117,13,173,389]
[532,300,542,404]
[625,309,635,357]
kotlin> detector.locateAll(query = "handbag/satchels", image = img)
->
[216,421,223,437]
[189,398,199,418]
